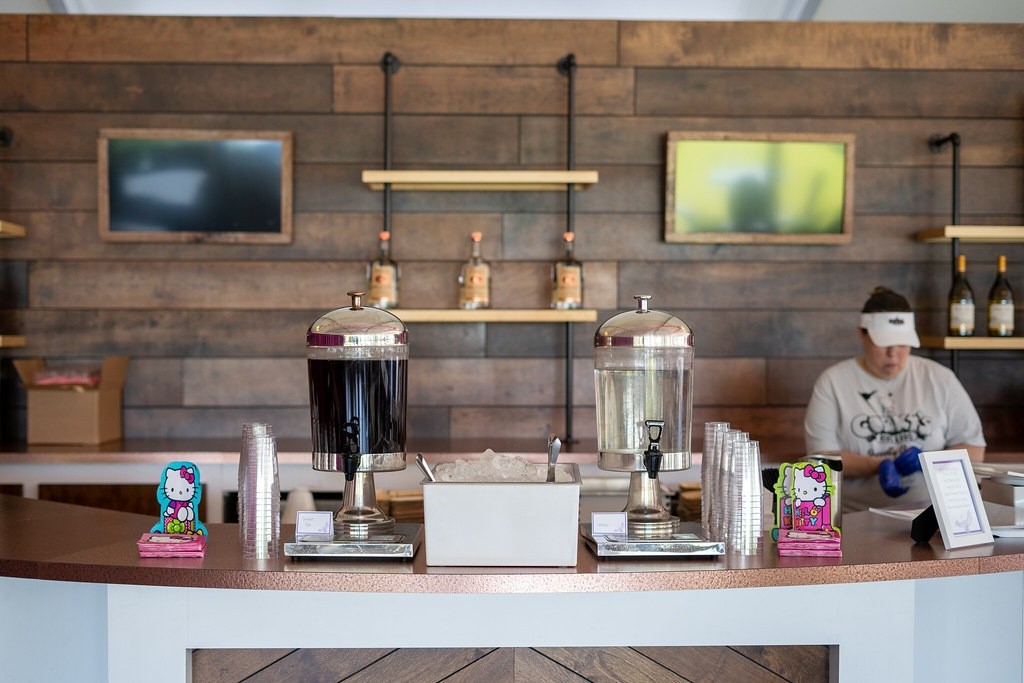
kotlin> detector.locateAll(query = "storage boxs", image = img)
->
[12,354,130,447]
[418,463,584,569]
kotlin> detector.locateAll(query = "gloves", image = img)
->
[880,459,909,497]
[894,447,924,477]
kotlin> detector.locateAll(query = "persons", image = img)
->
[806,285,987,514]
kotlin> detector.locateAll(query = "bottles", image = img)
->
[987,256,1015,337]
[550,232,583,310]
[367,231,399,309]
[458,233,490,308]
[948,253,976,336]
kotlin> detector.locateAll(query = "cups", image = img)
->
[235,422,282,560]
[800,452,843,528]
[700,422,764,556]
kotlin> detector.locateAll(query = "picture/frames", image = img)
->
[660,128,857,247]
[94,126,297,244]
[912,448,995,549]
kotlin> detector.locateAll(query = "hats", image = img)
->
[858,312,921,348]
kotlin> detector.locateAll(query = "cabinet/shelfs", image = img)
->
[912,226,1024,350]
[0,220,27,347]
[361,167,599,323]
